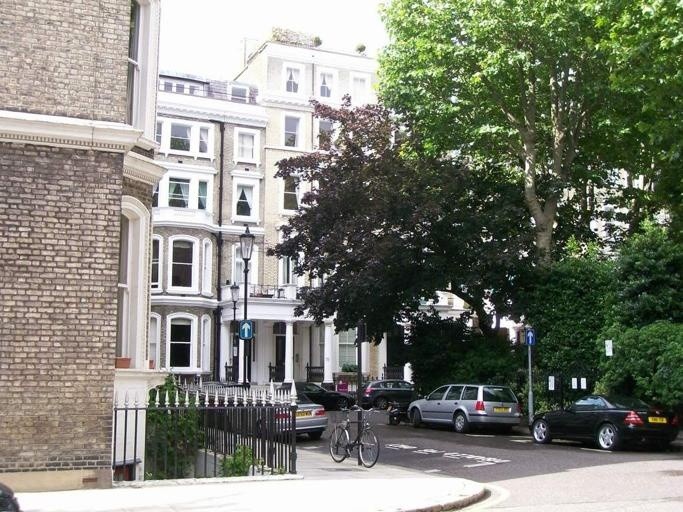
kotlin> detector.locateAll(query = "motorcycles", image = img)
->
[384,394,426,428]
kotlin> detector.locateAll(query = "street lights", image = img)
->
[225,280,242,383]
[238,225,256,389]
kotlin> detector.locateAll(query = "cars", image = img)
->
[270,380,356,413]
[405,384,519,433]
[528,392,679,451]
[249,387,331,443]
[356,379,414,412]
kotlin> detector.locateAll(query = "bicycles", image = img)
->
[328,407,384,469]
